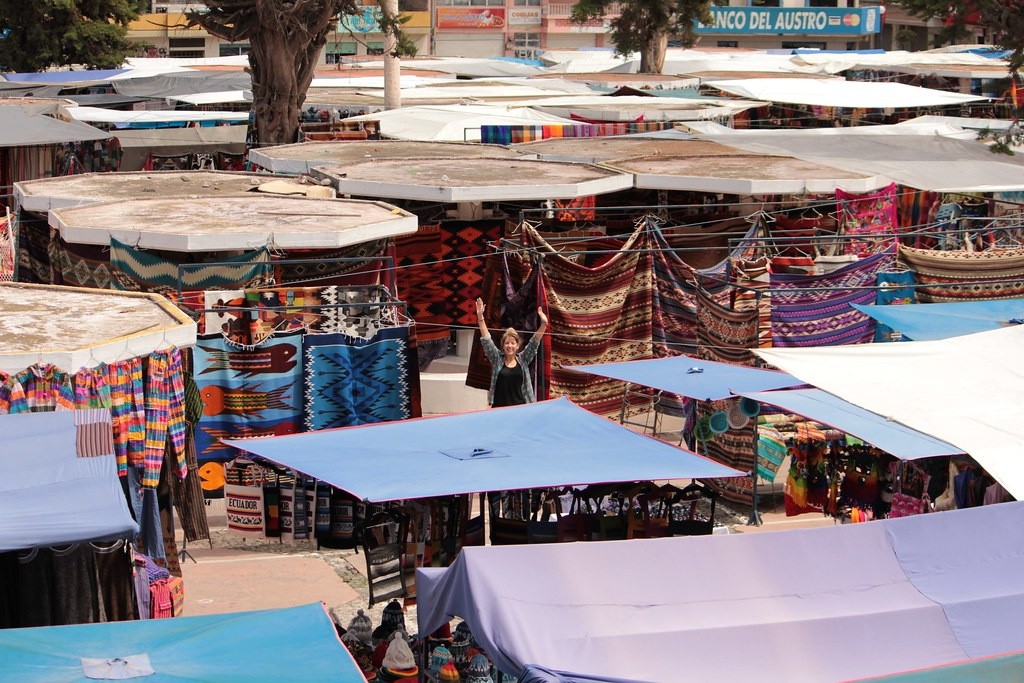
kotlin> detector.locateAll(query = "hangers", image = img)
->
[0,60,1024,683]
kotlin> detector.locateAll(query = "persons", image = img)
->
[475,298,548,413]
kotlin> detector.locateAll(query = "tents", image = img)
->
[1,405,139,632]
[416,492,1024,683]
[1,36,1024,200]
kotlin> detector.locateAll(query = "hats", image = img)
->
[694,397,760,442]
[327,599,518,683]
